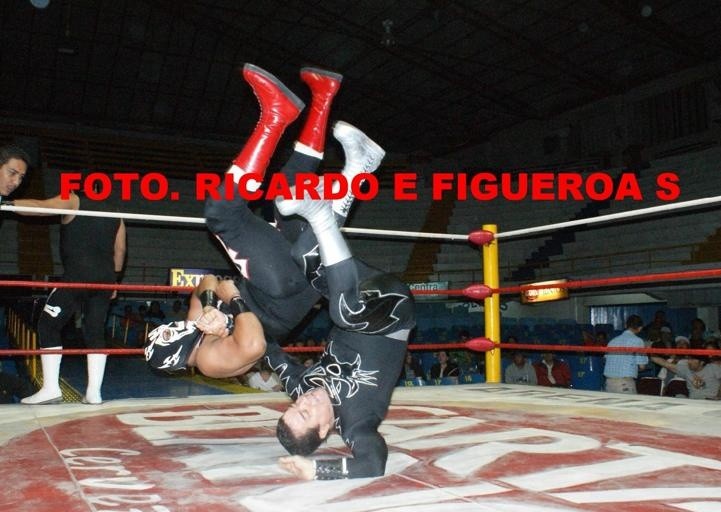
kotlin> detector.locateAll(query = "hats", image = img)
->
[675,335,691,348]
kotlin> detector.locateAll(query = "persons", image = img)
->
[0,139,129,404]
[120,62,421,482]
[124,281,721,405]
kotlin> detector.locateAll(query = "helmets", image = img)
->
[144,320,204,375]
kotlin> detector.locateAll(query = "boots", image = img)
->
[229,64,342,183]
[275,119,387,268]
[82,354,108,405]
[20,346,64,405]
